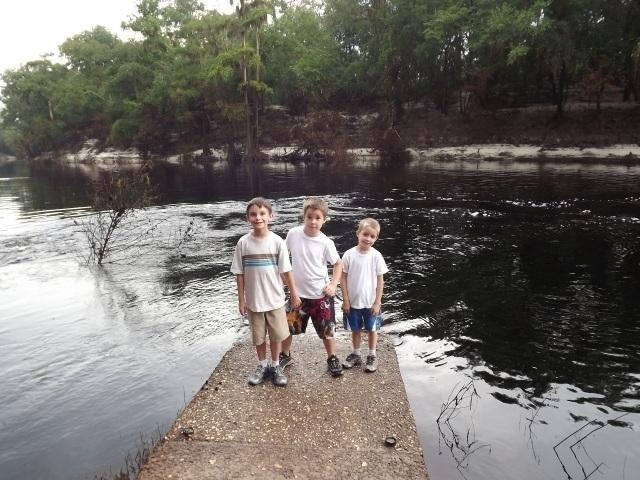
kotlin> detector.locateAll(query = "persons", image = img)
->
[228,196,303,386]
[278,196,343,377]
[337,217,390,374]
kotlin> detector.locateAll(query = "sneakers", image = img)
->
[248,362,269,386]
[326,354,342,376]
[270,365,287,386]
[279,351,293,372]
[341,353,362,369]
[365,355,379,372]
